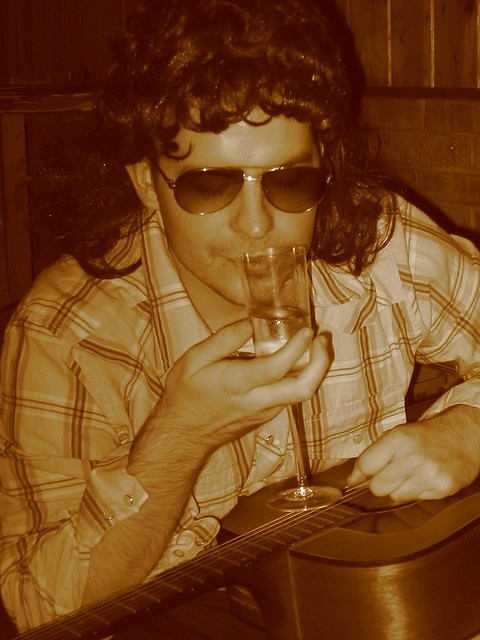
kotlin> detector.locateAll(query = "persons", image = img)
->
[1,0,479,635]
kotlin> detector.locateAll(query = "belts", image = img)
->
[242,244,343,512]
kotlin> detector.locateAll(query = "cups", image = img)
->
[141,147,333,216]
